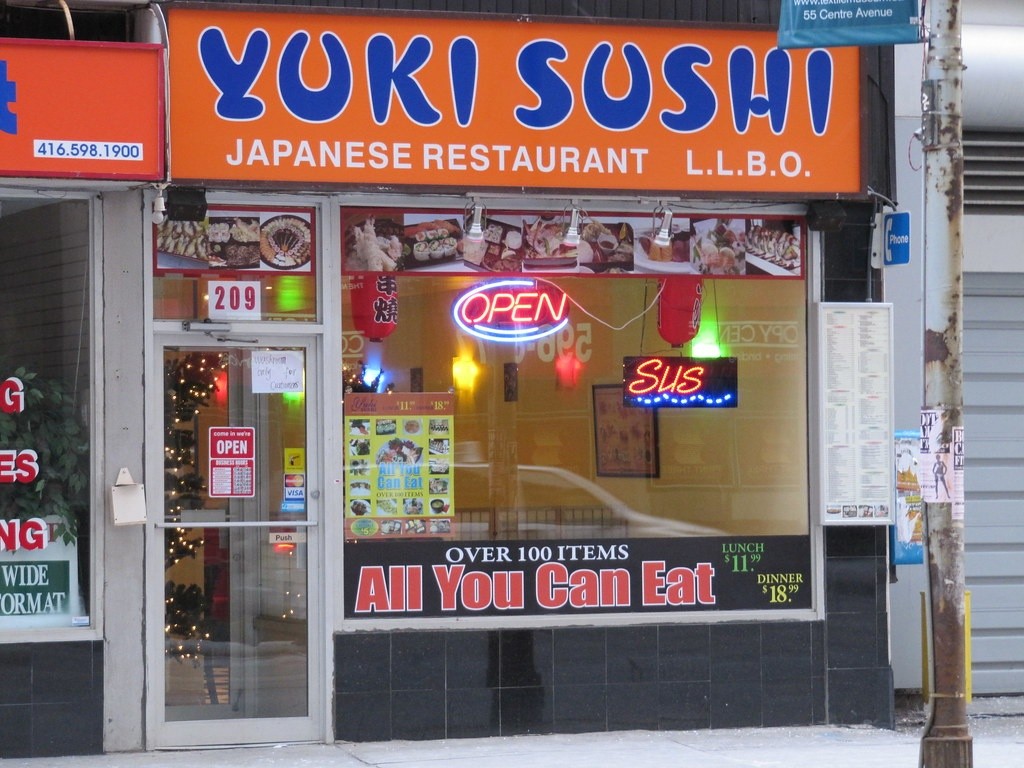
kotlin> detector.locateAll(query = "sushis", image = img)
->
[208,222,231,243]
[741,226,803,270]
[698,223,744,269]
[403,219,457,261]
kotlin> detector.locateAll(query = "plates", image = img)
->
[351,518,378,536]
[580,266,595,274]
[350,499,372,516]
[634,226,690,274]
[259,215,311,270]
[404,218,464,270]
[403,417,423,435]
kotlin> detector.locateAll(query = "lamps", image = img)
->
[805,201,847,232]
[555,205,589,246]
[166,185,207,222]
[649,205,675,246]
[463,201,488,241]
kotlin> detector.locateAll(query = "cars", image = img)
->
[223,459,740,650]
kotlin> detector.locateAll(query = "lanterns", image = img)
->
[656,275,704,348]
[348,275,400,342]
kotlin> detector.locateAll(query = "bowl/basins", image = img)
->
[431,499,444,513]
[429,239,445,260]
[597,233,619,255]
[413,241,429,261]
[444,237,458,256]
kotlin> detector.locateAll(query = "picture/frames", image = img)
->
[592,383,660,479]
